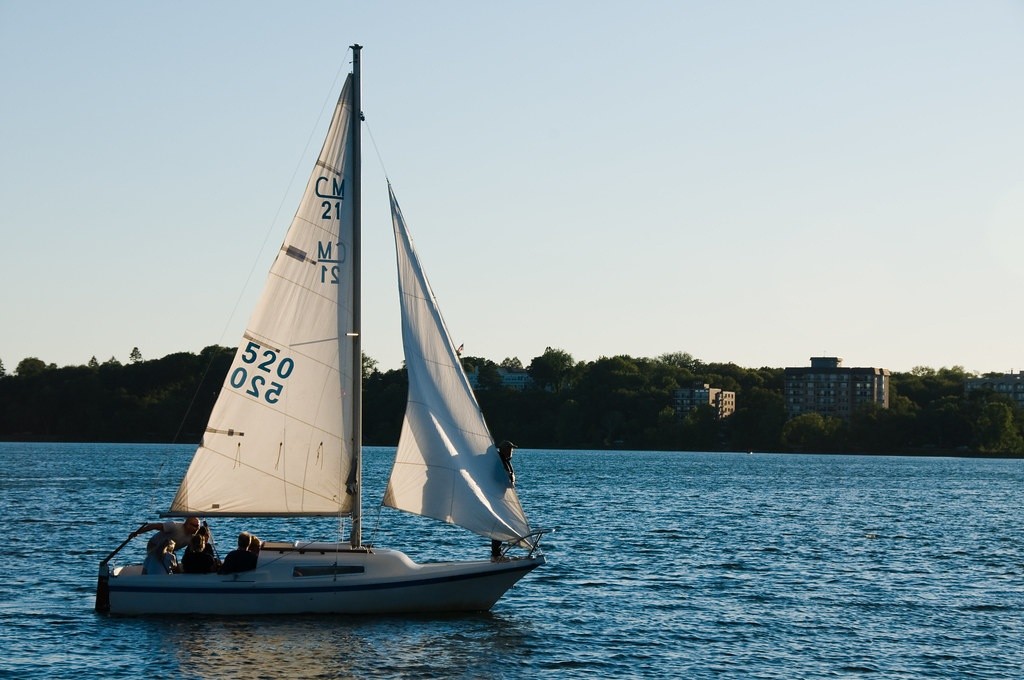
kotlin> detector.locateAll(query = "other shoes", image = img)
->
[491,555,510,562]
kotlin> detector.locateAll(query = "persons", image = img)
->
[491,441,518,562]
[142,537,178,574]
[181,520,223,567]
[129,516,200,574]
[217,533,260,574]
[183,535,218,574]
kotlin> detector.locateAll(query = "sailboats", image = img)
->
[94,42,555,622]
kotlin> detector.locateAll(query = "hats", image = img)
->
[499,440,518,449]
